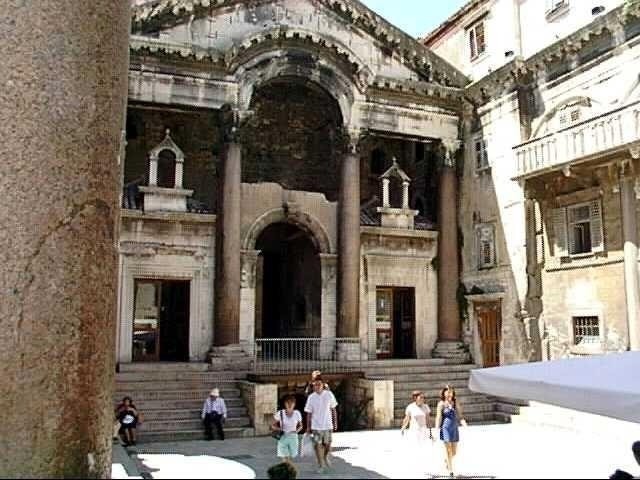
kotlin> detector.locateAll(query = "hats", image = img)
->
[208,388,221,396]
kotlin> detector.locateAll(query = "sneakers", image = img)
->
[312,457,333,474]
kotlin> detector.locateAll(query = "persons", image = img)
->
[305,370,330,393]
[401,389,432,452]
[304,376,338,473]
[270,395,304,464]
[267,459,299,480]
[434,383,468,476]
[115,396,140,445]
[201,386,229,442]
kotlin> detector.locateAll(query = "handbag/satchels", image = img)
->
[272,430,281,440]
